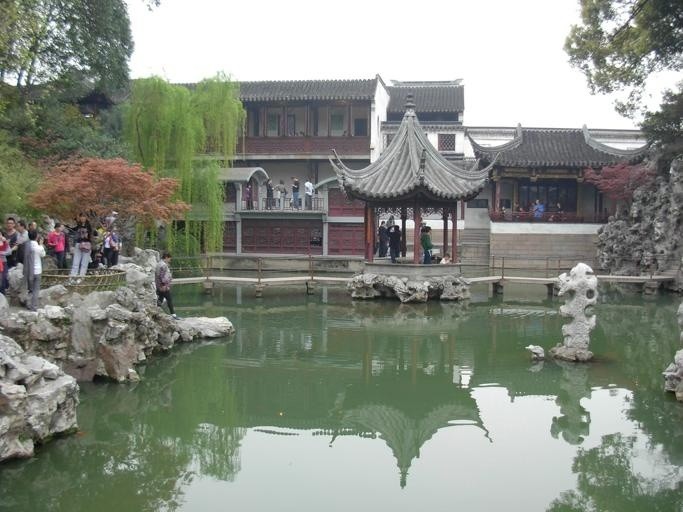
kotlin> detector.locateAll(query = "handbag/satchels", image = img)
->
[80,242,91,252]
[156,279,170,292]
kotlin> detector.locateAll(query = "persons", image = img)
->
[288,177,299,210]
[273,180,288,210]
[501,199,564,222]
[265,179,274,210]
[378,214,433,264]
[155,253,181,320]
[244,185,254,210]
[287,129,359,136]
[303,178,313,210]
[439,254,450,264]
[0,212,123,312]
